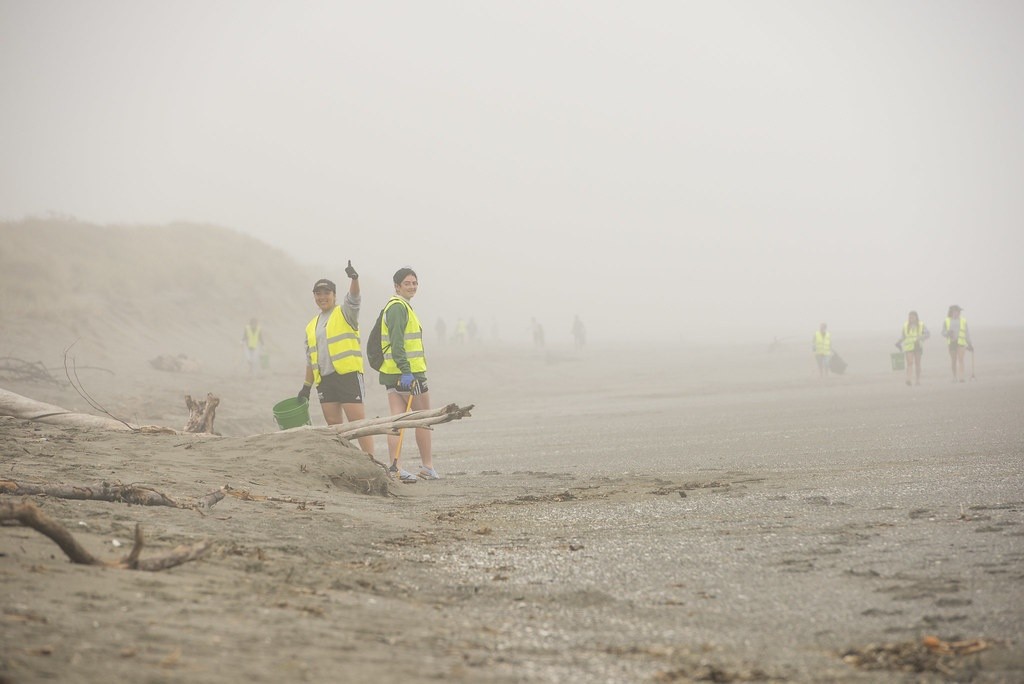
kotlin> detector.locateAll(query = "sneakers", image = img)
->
[418,465,439,480]
[398,468,417,483]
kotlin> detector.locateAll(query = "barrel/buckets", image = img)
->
[890,345,904,370]
[272,396,312,430]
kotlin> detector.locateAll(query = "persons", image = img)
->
[897,311,929,386]
[378,268,439,483]
[297,260,376,460]
[242,317,262,370]
[942,305,974,382]
[813,322,835,378]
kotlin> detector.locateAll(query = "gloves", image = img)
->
[399,373,415,391]
[298,385,311,401]
[345,260,358,279]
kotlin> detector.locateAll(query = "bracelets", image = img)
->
[304,381,312,387]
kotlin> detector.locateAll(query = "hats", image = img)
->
[312,279,336,292]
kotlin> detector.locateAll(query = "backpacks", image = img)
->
[367,299,409,371]
